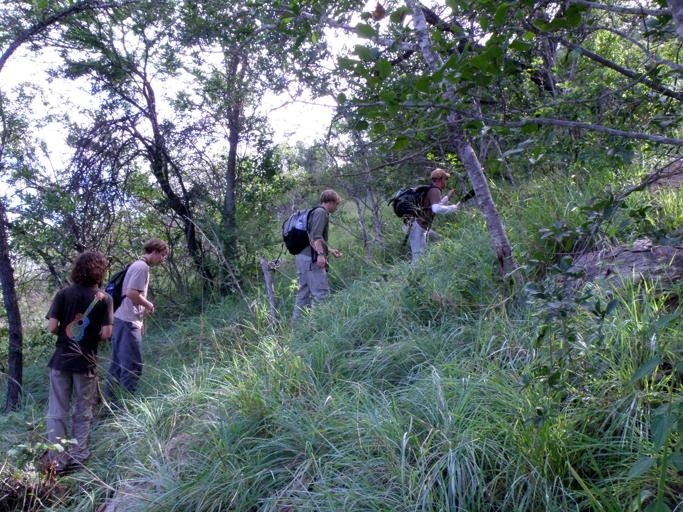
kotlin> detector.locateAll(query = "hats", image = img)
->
[431,168,450,179]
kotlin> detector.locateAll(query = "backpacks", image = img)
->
[104,262,133,312]
[282,206,324,255]
[389,185,436,221]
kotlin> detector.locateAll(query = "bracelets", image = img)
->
[317,250,326,255]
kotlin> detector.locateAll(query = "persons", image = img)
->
[41,248,114,478]
[101,236,170,416]
[291,187,343,319]
[396,168,466,263]
